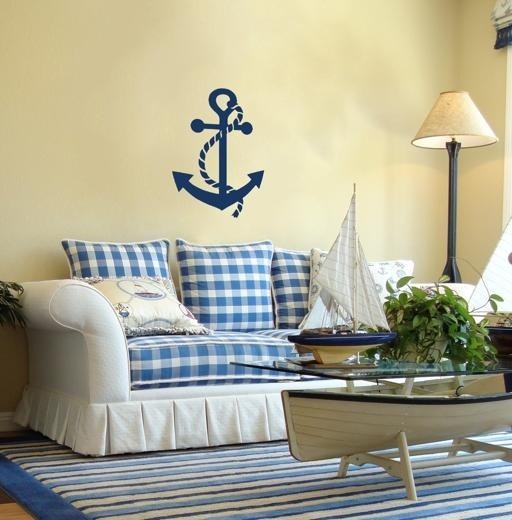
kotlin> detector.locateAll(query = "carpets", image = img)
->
[1,435,512,520]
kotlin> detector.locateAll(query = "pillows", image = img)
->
[272,245,312,330]
[297,248,414,330]
[60,238,173,278]
[173,238,277,332]
[71,276,214,337]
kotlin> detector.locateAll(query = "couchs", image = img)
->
[9,277,478,461]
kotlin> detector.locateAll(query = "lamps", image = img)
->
[410,90,499,283]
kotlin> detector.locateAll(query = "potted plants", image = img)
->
[356,276,505,374]
